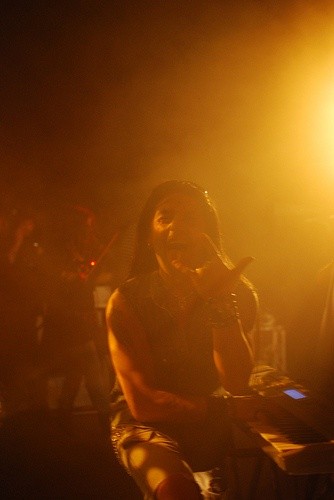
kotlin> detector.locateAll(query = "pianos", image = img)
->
[236,364,334,475]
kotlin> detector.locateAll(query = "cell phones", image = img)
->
[282,386,309,401]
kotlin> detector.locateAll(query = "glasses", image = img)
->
[153,181,208,201]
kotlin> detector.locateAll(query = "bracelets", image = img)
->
[205,291,240,329]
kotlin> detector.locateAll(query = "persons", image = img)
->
[101,179,289,500]
[6,134,118,415]
